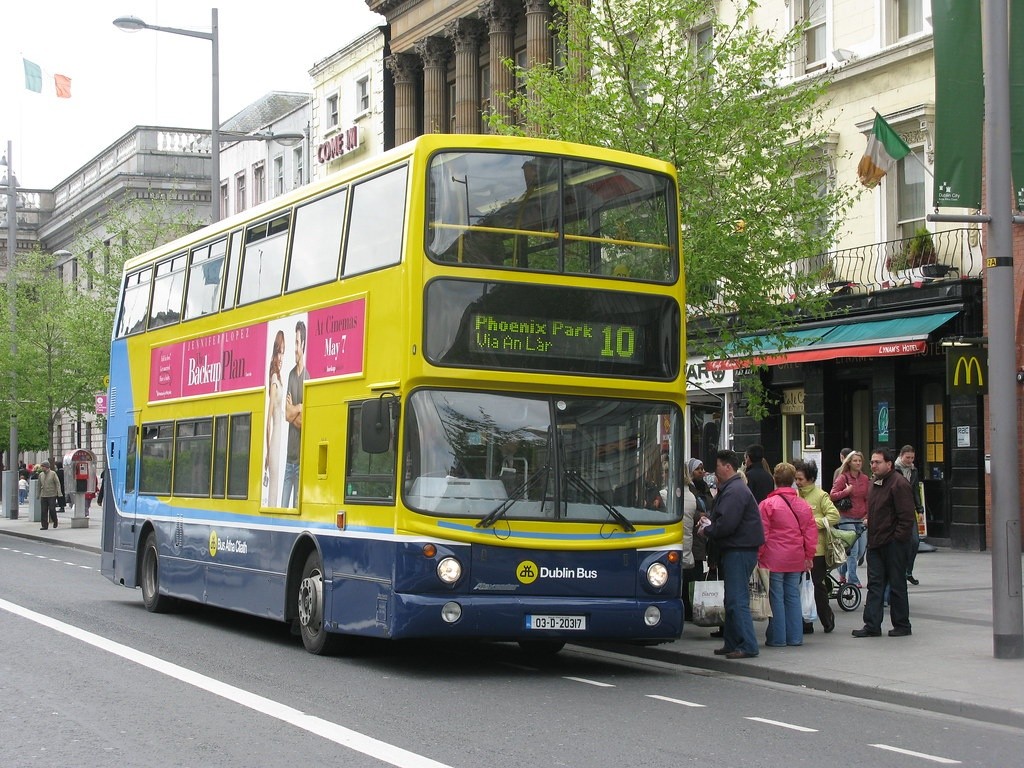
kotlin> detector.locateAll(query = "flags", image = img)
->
[857,112,913,189]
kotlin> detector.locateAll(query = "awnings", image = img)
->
[703,311,962,371]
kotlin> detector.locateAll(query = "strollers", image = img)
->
[822,521,867,612]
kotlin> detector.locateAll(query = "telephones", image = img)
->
[76,462,88,480]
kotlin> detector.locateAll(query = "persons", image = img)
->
[35,460,62,530]
[18,461,41,505]
[55,462,66,512]
[85,476,99,518]
[265,330,285,508]
[281,321,311,508]
[403,416,468,479]
[792,459,841,635]
[646,443,774,637]
[757,462,818,647]
[851,446,916,637]
[476,432,532,480]
[830,446,924,588]
[695,449,765,658]
[97,469,104,507]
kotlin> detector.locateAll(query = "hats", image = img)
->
[40,460,50,469]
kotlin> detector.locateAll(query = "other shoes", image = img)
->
[837,567,846,583]
[711,630,724,637]
[40,527,47,530]
[56,506,64,512]
[852,624,882,637]
[53,519,58,528]
[888,628,911,636]
[824,612,835,633]
[803,619,813,634]
[906,576,919,585]
[854,583,862,588]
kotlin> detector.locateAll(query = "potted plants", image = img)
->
[887,227,950,287]
[828,277,851,295]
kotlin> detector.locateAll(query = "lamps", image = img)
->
[832,48,858,62]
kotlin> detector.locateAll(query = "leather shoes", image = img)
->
[725,650,754,658]
[714,645,734,654]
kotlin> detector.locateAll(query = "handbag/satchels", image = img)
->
[833,473,852,512]
[749,560,773,622]
[799,569,817,623]
[826,539,848,567]
[689,566,726,627]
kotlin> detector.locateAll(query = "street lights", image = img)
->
[112,8,305,225]
[7,250,73,520]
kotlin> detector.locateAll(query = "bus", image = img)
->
[101,132,724,659]
